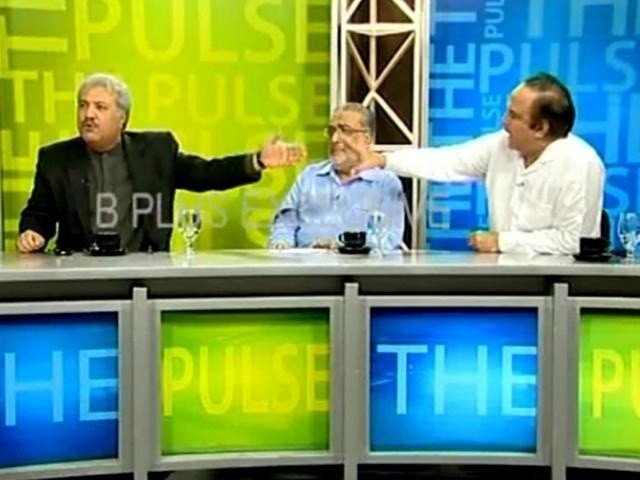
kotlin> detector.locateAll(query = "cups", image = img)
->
[96,232,120,250]
[579,237,604,254]
[337,231,366,247]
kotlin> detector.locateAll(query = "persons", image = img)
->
[337,75,605,256]
[15,75,308,255]
[266,103,407,251]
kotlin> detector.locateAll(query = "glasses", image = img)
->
[324,123,368,136]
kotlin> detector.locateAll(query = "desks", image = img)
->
[1,249,639,480]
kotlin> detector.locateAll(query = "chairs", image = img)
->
[600,211,610,237]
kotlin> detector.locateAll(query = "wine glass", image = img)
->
[617,212,639,262]
[179,209,202,258]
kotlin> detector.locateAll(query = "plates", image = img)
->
[338,246,371,254]
[573,252,612,261]
